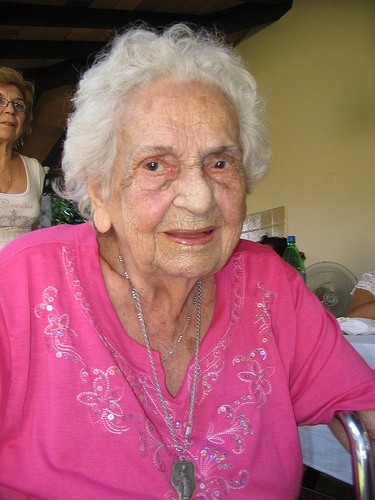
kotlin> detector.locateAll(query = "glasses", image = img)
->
[0,98,27,112]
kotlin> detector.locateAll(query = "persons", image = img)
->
[346,269,375,319]
[0,66,45,249]
[0,20,375,499]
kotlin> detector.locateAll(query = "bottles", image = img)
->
[282,236,306,282]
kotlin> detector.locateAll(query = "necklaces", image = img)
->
[112,246,217,500]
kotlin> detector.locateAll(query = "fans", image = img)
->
[305,261,359,318]
